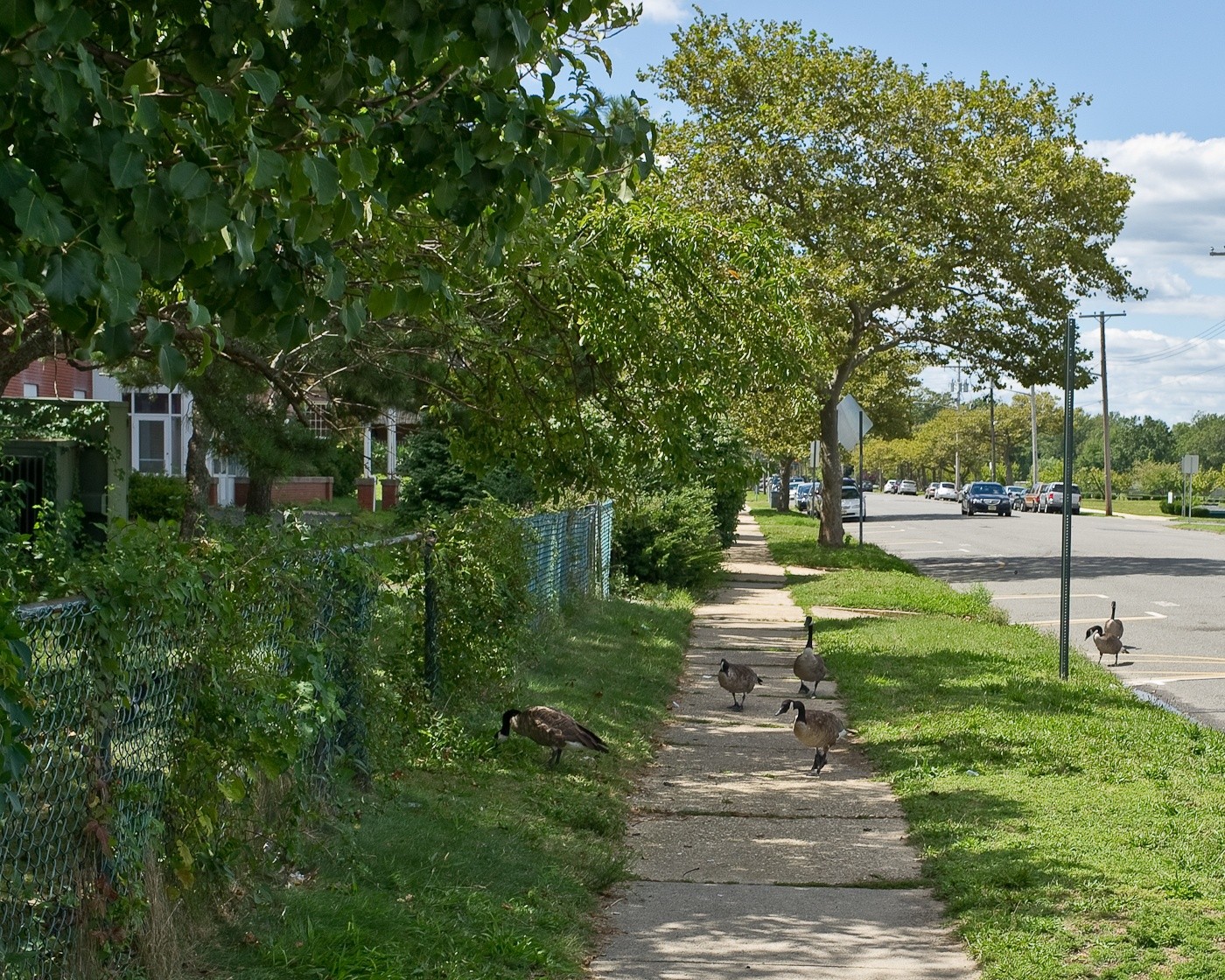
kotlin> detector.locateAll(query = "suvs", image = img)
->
[1038,482,1082,514]
[1021,482,1049,512]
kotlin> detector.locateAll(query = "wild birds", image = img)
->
[774,699,846,774]
[1084,600,1131,667]
[493,705,611,767]
[717,657,764,710]
[793,615,828,699]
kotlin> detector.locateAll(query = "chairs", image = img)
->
[848,491,854,496]
[974,487,980,493]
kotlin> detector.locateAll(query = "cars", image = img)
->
[760,474,860,517]
[841,486,867,519]
[960,482,1012,516]
[1004,485,1029,512]
[884,479,903,494]
[862,480,873,493]
[924,482,937,499]
[896,479,917,495]
[956,483,971,503]
[934,482,958,502]
